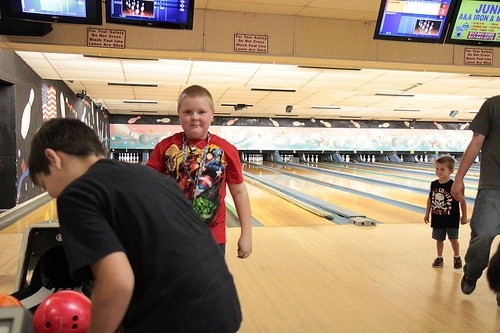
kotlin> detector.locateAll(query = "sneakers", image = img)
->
[489,280,500,309]
[460,274,478,295]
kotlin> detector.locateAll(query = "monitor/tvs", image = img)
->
[15,0,102,25]
[445,0,500,46]
[372,0,456,44]
[106,0,195,31]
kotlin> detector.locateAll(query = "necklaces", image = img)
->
[181,133,211,185]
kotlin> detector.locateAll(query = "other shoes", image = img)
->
[453,256,462,269]
[432,257,444,268]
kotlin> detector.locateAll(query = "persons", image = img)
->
[423,155,468,269]
[450,94,500,296]
[145,84,253,260]
[28,116,243,333]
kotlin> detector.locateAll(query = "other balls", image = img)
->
[0,293,23,307]
[34,290,92,333]
